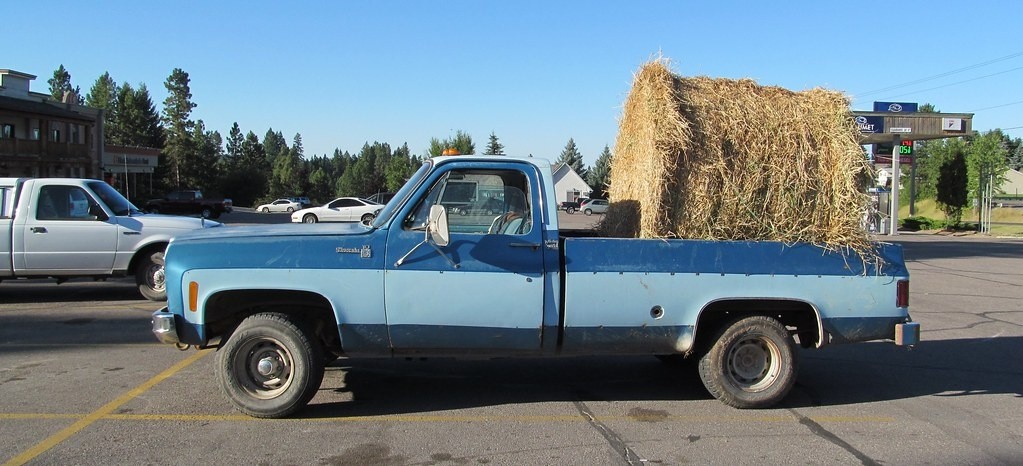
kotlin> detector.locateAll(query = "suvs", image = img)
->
[580,198,610,216]
[69,188,89,218]
[290,197,310,206]
[367,193,430,227]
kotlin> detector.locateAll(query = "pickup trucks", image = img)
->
[158,149,924,420]
[0,177,225,302]
[560,197,589,214]
[143,189,234,220]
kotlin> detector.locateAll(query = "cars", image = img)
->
[452,197,504,217]
[292,197,387,223]
[256,198,303,213]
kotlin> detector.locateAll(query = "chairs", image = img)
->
[37,196,58,218]
[486,211,531,235]
